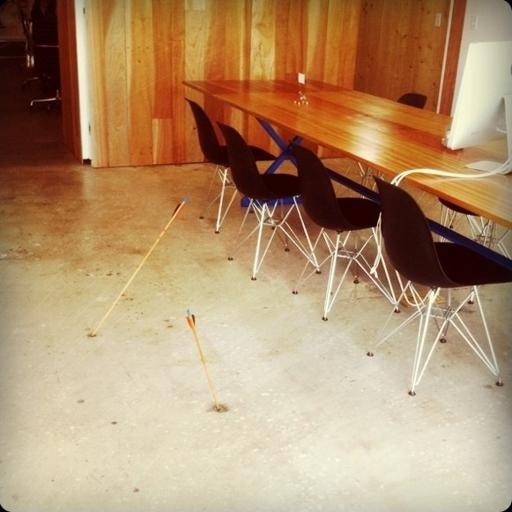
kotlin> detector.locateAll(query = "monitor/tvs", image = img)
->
[443,0,512,174]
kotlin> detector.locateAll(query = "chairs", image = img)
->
[217,122,321,281]
[290,141,400,321]
[29,27,62,106]
[437,196,485,242]
[184,96,284,231]
[345,93,427,199]
[367,171,512,397]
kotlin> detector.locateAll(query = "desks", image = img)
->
[182,80,512,230]
